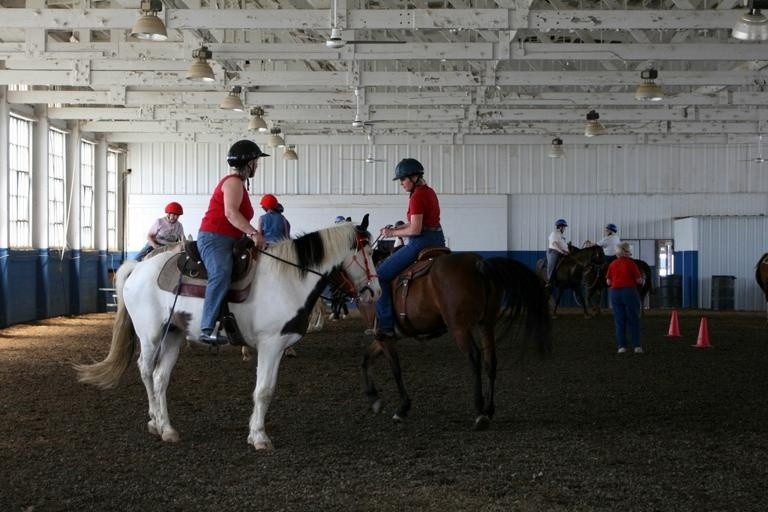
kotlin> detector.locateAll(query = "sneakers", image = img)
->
[197,329,229,344]
[364,325,401,340]
[633,345,645,354]
[615,347,627,353]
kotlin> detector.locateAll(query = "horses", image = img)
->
[327,250,553,430]
[71,214,383,450]
[567,241,652,317]
[535,246,608,320]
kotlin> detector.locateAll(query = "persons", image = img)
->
[586,223,621,305]
[334,215,345,224]
[255,194,284,249]
[275,203,290,238]
[371,157,445,338]
[197,138,272,344]
[544,219,568,287]
[606,242,647,354]
[133,201,183,262]
[390,221,409,255]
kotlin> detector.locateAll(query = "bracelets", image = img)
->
[246,229,258,238]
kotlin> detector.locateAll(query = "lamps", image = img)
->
[584,113,608,138]
[218,86,244,112]
[267,126,287,148]
[731,0,768,43]
[184,46,216,83]
[128,1,170,42]
[546,138,567,158]
[633,69,665,105]
[281,145,299,161]
[247,106,268,132]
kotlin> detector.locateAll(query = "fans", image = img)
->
[332,116,384,128]
[737,154,768,163]
[297,31,407,51]
[337,154,391,166]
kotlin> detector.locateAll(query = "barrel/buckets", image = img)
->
[660,275,682,309]
[710,275,736,310]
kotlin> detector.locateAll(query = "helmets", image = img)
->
[225,138,271,168]
[164,201,184,215]
[276,204,284,213]
[259,193,278,209]
[394,220,405,228]
[606,222,618,233]
[391,158,425,182]
[554,217,569,228]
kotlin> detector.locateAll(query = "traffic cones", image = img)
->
[663,310,684,338]
[691,316,713,348]
